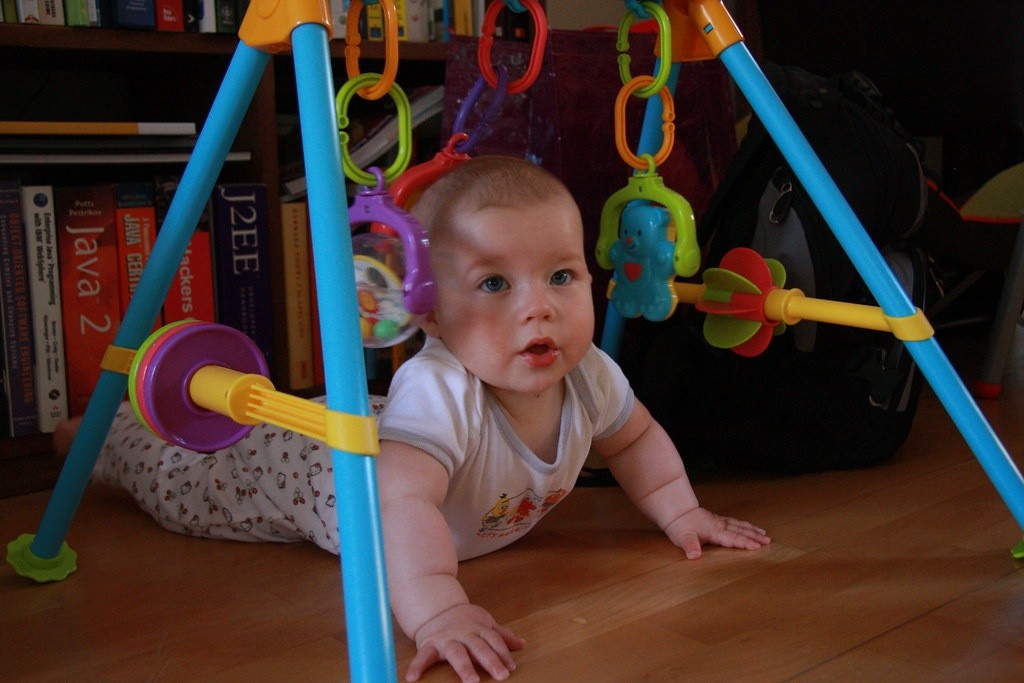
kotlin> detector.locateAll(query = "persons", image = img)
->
[47,156,769,683]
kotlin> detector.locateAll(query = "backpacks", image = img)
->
[609,61,942,479]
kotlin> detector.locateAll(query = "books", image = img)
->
[0,86,447,437]
[0,0,524,43]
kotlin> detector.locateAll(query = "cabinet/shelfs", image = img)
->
[0,0,550,498]
